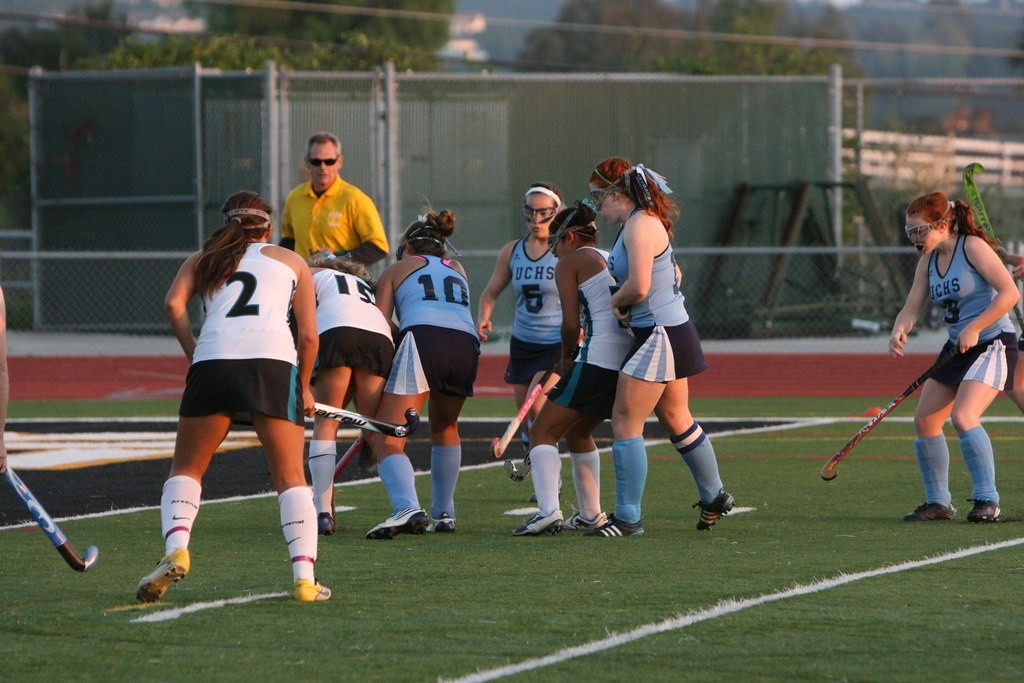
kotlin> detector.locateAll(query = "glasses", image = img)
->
[522,204,558,223]
[308,155,339,166]
[547,235,560,254]
[590,188,608,210]
[905,221,933,243]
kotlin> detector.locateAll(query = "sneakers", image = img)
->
[904,501,957,524]
[692,488,735,531]
[365,507,429,540]
[424,512,456,533]
[135,547,191,604]
[967,498,1001,522]
[563,511,608,530]
[583,512,645,538]
[294,577,331,602]
[317,512,335,536]
[512,508,564,536]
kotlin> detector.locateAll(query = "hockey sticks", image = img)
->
[334,431,366,476]
[490,354,565,459]
[821,343,963,481]
[961,162,1024,330]
[5,466,99,571]
[503,455,531,482]
[312,401,421,439]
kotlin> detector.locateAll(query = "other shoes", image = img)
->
[527,480,562,503]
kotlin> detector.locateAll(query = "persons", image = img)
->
[291,251,396,534]
[888,192,1020,522]
[588,156,736,537]
[0,288,9,475]
[475,183,584,503]
[137,191,330,604]
[279,131,389,267]
[992,244,1024,413]
[376,209,479,539]
[512,197,635,536]
